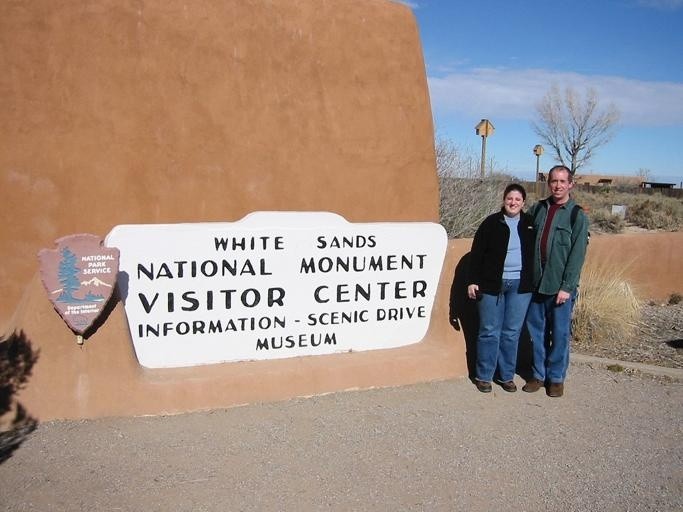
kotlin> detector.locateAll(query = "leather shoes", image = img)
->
[476,381,492,392]
[494,380,517,392]
[547,383,564,397]
[522,379,546,393]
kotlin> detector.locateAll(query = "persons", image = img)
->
[522,164,591,398]
[466,183,535,393]
